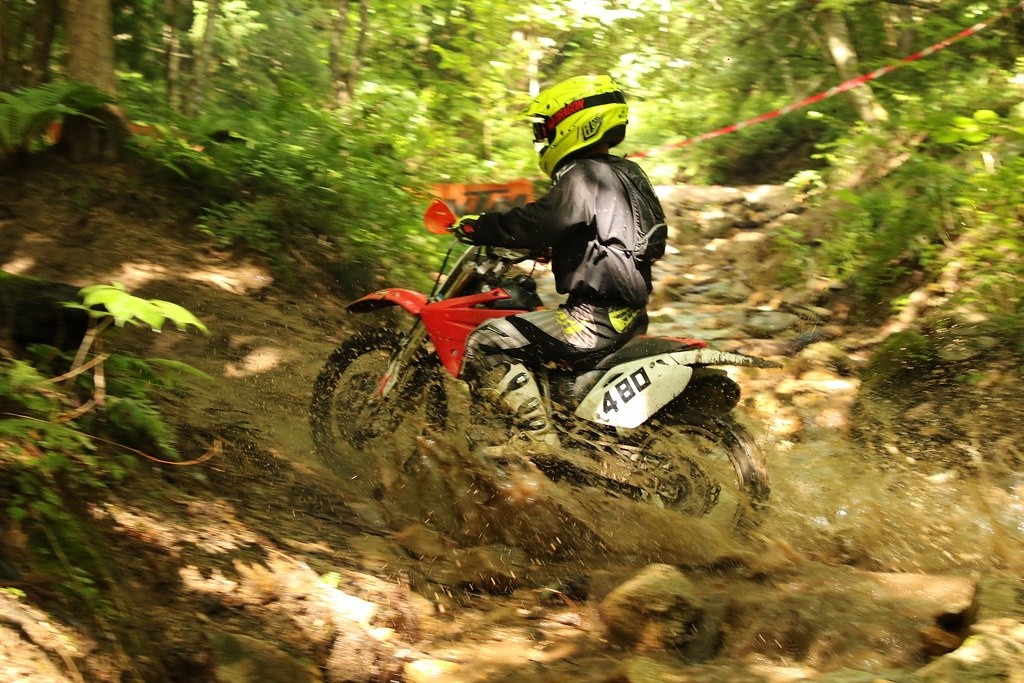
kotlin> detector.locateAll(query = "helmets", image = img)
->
[525,74,628,180]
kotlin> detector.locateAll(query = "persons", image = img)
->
[448,75,668,465]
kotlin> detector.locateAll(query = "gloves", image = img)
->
[453,213,480,245]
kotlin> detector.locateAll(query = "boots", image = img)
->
[485,361,558,462]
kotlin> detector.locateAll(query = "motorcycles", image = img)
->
[307,197,772,545]
